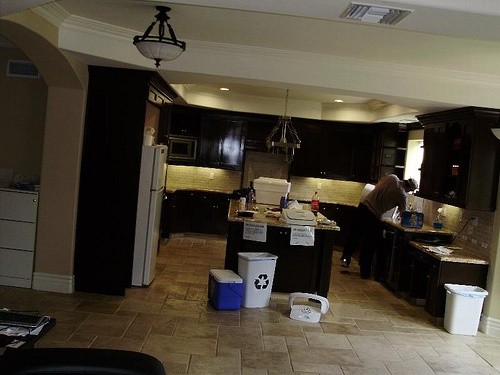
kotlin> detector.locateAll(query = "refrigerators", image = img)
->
[131,145,169,287]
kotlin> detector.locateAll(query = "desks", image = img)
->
[224,199,340,301]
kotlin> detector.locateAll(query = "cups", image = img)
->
[239,197,246,210]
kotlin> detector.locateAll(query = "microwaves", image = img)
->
[167,139,195,159]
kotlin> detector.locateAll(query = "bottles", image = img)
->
[279,196,286,211]
[311,192,320,216]
[248,189,254,204]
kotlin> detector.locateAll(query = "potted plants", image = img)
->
[433,207,447,229]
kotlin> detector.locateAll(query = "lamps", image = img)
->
[265,88,301,159]
[133,6,187,69]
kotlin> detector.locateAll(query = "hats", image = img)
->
[407,178,416,190]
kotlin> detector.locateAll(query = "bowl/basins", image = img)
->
[434,222,443,230]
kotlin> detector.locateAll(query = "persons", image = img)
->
[340,174,418,279]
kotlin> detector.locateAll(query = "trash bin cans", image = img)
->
[237,252,279,309]
[443,283,489,336]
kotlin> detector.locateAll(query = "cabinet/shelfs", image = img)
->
[154,102,500,320]
[0,188,39,289]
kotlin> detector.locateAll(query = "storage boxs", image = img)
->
[209,269,243,311]
[401,211,424,228]
